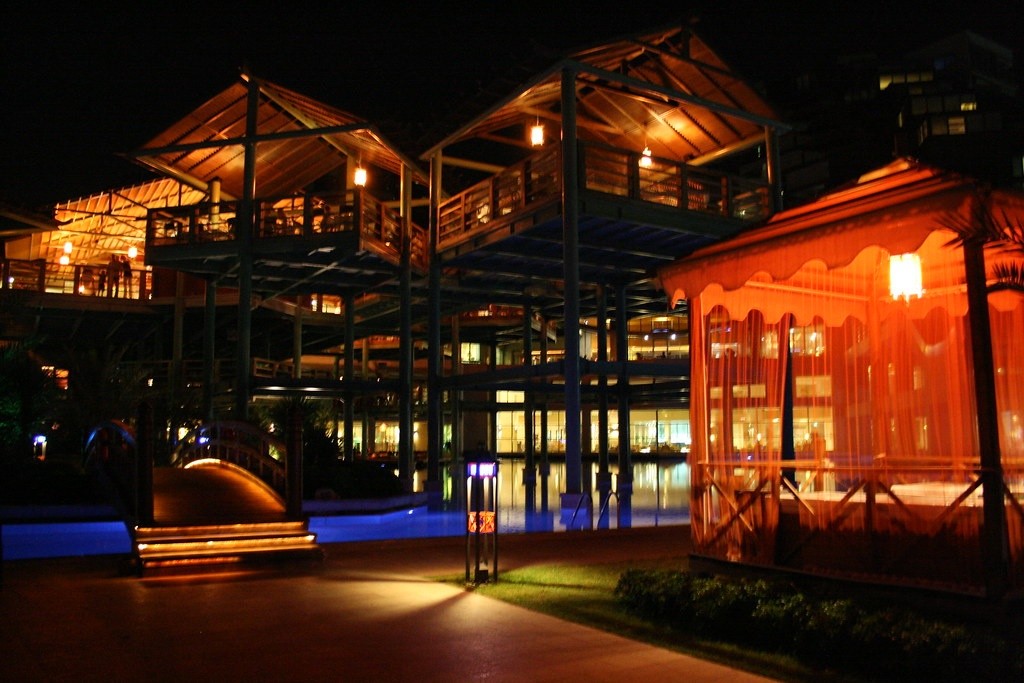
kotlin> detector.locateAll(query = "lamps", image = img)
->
[530,105,545,146]
[641,136,652,167]
[354,158,367,187]
[889,252,923,303]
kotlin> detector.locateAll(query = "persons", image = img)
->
[79,252,132,299]
